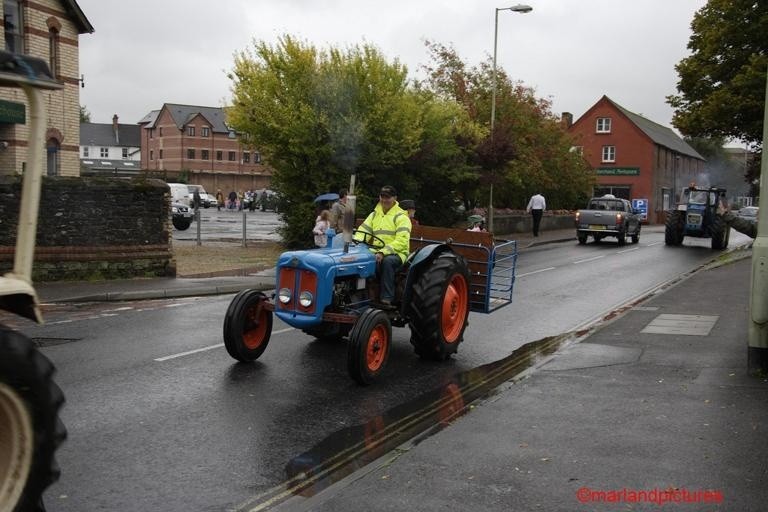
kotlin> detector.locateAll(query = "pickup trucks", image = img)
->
[574,197,641,245]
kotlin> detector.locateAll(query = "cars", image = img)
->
[172,182,218,230]
[738,207,759,223]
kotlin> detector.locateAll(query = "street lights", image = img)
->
[487,3,534,234]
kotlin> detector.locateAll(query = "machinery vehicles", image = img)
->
[664,186,730,250]
[222,223,518,386]
[0,47,67,512]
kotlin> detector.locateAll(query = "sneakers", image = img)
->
[381,299,392,306]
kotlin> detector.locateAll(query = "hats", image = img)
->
[400,200,416,210]
[379,185,397,199]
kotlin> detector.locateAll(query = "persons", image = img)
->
[526,189,547,237]
[311,188,352,248]
[716,199,759,239]
[215,187,267,212]
[399,199,419,225]
[466,214,487,233]
[204,191,209,201]
[354,184,413,305]
[192,187,200,212]
